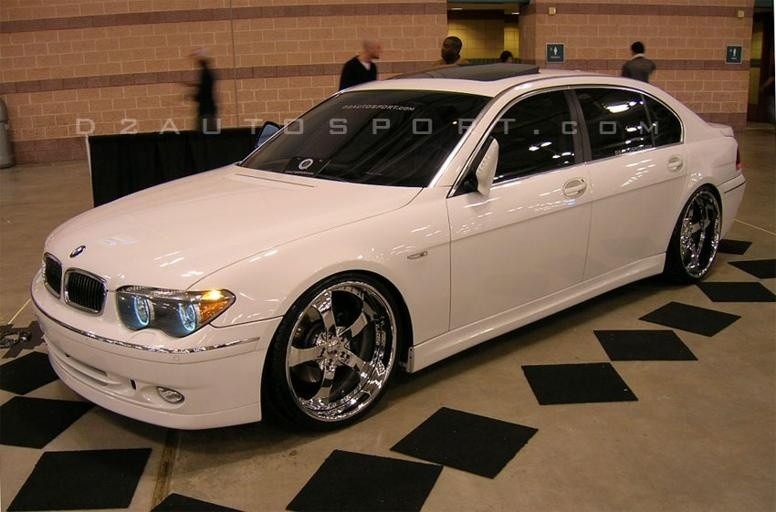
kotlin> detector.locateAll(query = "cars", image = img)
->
[31,63,745,429]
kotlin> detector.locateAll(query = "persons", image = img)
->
[339,34,381,91]
[183,50,217,132]
[622,42,655,83]
[500,51,512,64]
[430,37,469,66]
